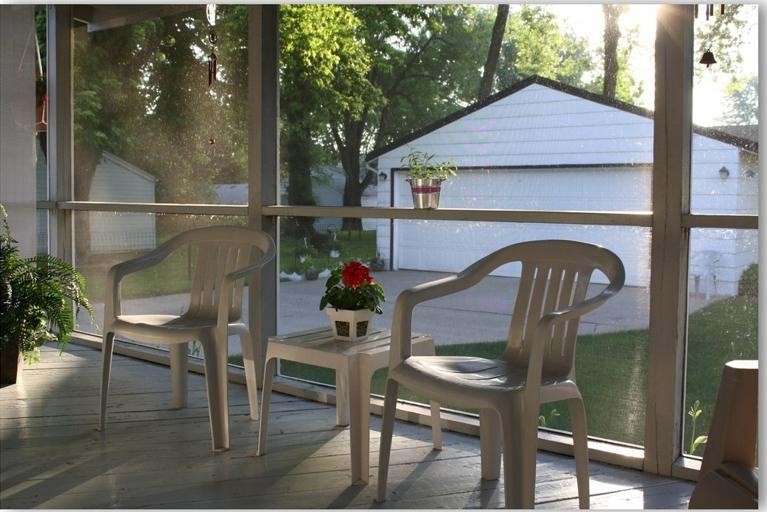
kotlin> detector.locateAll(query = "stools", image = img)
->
[255,325,442,487]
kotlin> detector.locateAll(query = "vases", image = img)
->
[9,96,47,134]
[324,306,374,342]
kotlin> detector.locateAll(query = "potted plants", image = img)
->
[399,143,458,209]
[0,202,94,391]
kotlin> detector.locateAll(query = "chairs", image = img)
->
[94,224,278,453]
[371,237,627,509]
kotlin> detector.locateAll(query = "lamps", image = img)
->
[718,166,729,179]
[378,170,387,182]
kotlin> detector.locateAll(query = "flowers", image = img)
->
[13,67,46,113]
[318,257,385,315]
